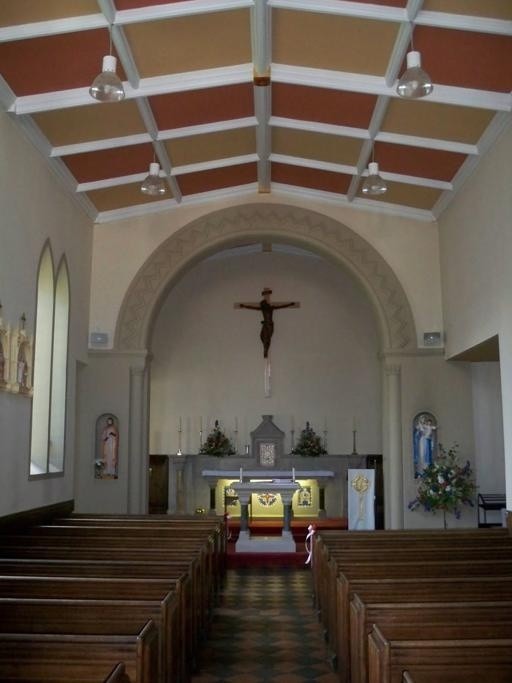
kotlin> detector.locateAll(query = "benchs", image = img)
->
[1,508,234,680]
[307,522,512,682]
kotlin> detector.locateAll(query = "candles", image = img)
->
[292,467,295,481]
[239,467,242,480]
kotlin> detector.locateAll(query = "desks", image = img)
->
[226,481,303,555]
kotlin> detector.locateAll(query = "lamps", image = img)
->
[90,20,168,198]
[361,7,436,198]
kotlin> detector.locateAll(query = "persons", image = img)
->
[414,417,439,471]
[98,416,118,476]
[240,299,297,358]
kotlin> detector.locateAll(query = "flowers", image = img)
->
[198,418,237,458]
[407,439,481,530]
[287,418,329,458]
